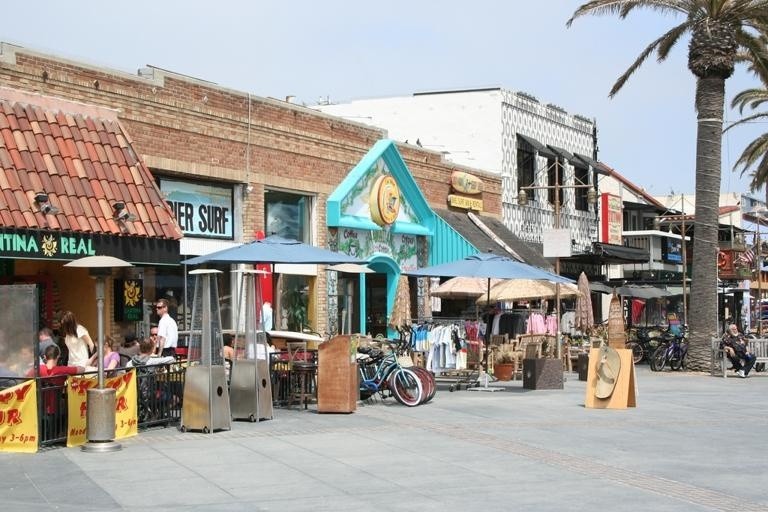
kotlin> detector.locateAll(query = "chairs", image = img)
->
[711,337,749,379]
[286,341,318,411]
[514,341,541,382]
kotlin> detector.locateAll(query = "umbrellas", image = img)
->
[575,271,594,337]
[400,249,575,388]
[477,279,585,342]
[431,276,503,317]
[182,230,369,330]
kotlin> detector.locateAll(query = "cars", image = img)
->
[750,298,768,328]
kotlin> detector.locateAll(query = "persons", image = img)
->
[220,331,279,406]
[720,324,756,377]
[1,297,178,427]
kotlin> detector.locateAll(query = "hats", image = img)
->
[125,333,138,343]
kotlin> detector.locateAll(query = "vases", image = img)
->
[494,362,515,380]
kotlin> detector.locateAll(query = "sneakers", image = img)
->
[736,369,749,378]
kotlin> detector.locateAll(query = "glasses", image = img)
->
[154,306,165,309]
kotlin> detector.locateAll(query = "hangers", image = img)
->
[489,308,541,315]
[408,319,465,331]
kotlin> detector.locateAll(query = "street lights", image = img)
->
[651,192,695,328]
[734,216,768,339]
[516,155,598,355]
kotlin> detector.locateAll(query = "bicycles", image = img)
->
[626,321,690,373]
[739,320,768,373]
[355,324,438,406]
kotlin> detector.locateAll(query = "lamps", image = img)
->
[113,203,136,224]
[335,114,375,127]
[423,142,474,161]
[36,192,58,217]
